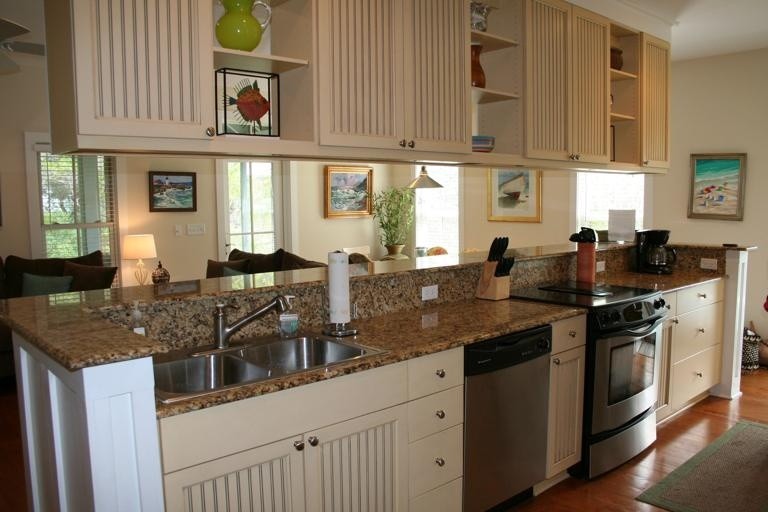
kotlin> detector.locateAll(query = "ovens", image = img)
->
[566,292,669,482]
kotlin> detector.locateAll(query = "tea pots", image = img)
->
[214,0,273,52]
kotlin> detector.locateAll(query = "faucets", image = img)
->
[212,295,288,350]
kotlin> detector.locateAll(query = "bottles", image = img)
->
[609,47,623,71]
[568,226,596,283]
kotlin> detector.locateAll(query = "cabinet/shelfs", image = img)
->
[533,313,586,496]
[160,360,409,511]
[674,279,724,420]
[410,347,464,511]
[42,0,670,174]
[656,290,679,428]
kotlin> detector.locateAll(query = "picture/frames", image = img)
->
[687,153,748,222]
[325,165,374,218]
[149,171,197,212]
[487,168,542,223]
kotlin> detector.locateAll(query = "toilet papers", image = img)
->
[327,250,351,324]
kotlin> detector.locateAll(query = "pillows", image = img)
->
[22,272,73,297]
[63,260,118,292]
[206,248,327,278]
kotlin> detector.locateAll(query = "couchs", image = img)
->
[1,250,103,395]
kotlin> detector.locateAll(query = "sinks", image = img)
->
[153,352,272,394]
[225,335,367,374]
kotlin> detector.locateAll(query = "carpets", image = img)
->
[635,419,768,512]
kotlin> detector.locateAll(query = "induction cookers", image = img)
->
[509,280,668,332]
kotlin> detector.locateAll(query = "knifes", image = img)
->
[487,236,515,276]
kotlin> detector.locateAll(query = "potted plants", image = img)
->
[372,187,414,256]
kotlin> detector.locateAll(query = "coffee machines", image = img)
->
[633,229,677,274]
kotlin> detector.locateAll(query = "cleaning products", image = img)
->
[279,294,299,339]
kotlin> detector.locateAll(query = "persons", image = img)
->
[701,185,716,194]
[716,181,731,191]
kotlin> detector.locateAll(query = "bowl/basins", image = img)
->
[472,134,495,153]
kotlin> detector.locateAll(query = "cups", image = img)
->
[413,247,427,258]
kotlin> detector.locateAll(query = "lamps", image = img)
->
[123,234,157,285]
[406,164,443,189]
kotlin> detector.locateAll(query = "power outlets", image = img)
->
[188,224,206,235]
[175,224,182,236]
